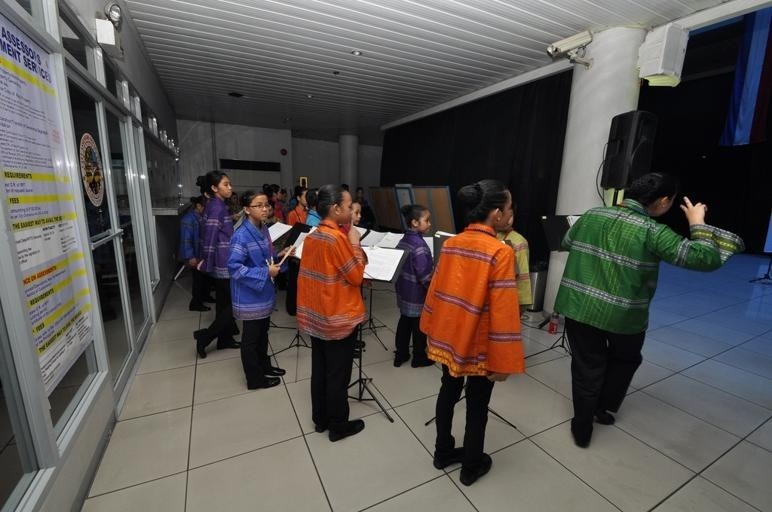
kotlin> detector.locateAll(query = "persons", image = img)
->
[180,171,376,357]
[497,202,532,317]
[552,173,746,449]
[394,205,435,367]
[418,179,524,486]
[223,190,296,389]
[295,185,368,442]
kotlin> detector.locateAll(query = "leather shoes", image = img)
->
[434,447,491,486]
[194,326,241,358]
[394,353,435,367]
[315,419,363,442]
[189,297,216,310]
[571,411,614,447]
[248,367,285,389]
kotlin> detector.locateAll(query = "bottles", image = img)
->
[548,312,559,333]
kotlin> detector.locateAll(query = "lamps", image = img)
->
[95,0,125,58]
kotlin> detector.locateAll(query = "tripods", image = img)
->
[523,317,573,359]
[425,384,516,429]
[347,282,394,423]
[359,282,388,351]
[268,261,312,358]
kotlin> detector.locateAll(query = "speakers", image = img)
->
[601,111,658,190]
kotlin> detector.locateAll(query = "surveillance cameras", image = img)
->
[546,30,593,58]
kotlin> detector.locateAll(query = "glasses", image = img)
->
[251,205,271,209]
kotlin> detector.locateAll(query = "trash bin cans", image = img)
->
[528,261,549,313]
